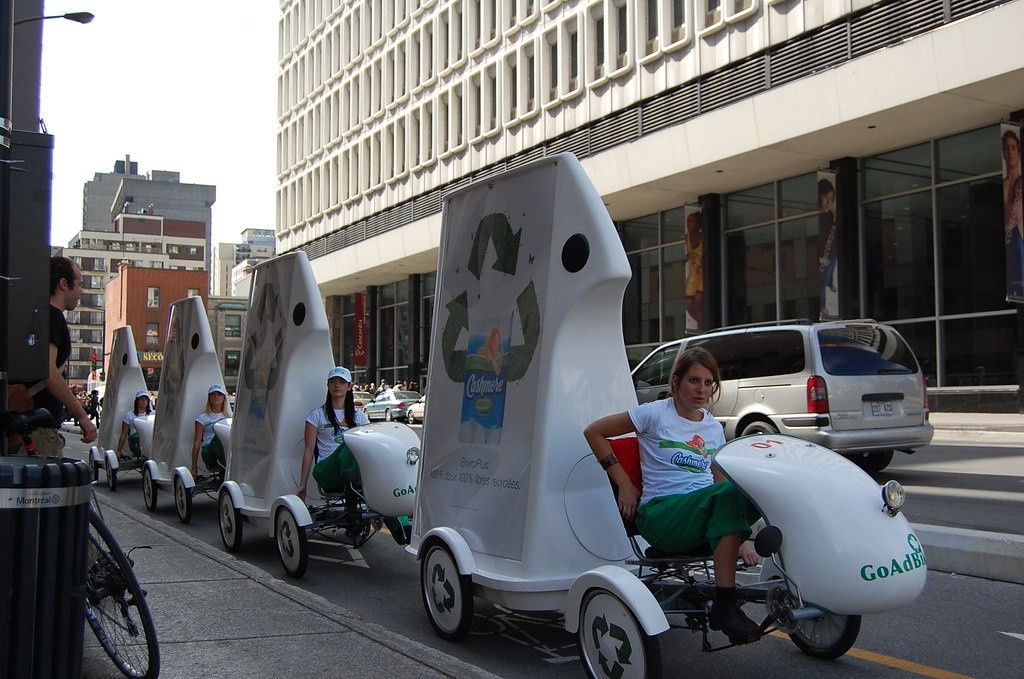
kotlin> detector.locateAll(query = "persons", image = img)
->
[583,348,765,645]
[5,257,99,458]
[351,379,420,395]
[295,365,414,545]
[1002,130,1024,301]
[818,179,837,318]
[117,389,156,461]
[65,384,104,430]
[191,384,235,483]
[684,210,704,327]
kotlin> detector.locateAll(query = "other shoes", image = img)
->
[404,525,412,544]
[708,602,762,643]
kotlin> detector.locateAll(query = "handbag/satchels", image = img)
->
[4,384,34,448]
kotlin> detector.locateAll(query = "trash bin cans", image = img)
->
[0,454,94,679]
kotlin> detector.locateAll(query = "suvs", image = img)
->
[630,316,935,473]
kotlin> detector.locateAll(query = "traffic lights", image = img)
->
[99,371,105,381]
[91,353,97,380]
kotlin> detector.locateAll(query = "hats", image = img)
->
[136,391,150,400]
[328,367,352,383]
[208,384,226,396]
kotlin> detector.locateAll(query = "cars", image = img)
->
[362,391,424,422]
[405,393,426,424]
[353,390,370,412]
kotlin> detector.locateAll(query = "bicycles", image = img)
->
[0,408,163,679]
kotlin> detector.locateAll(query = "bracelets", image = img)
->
[598,453,619,470]
